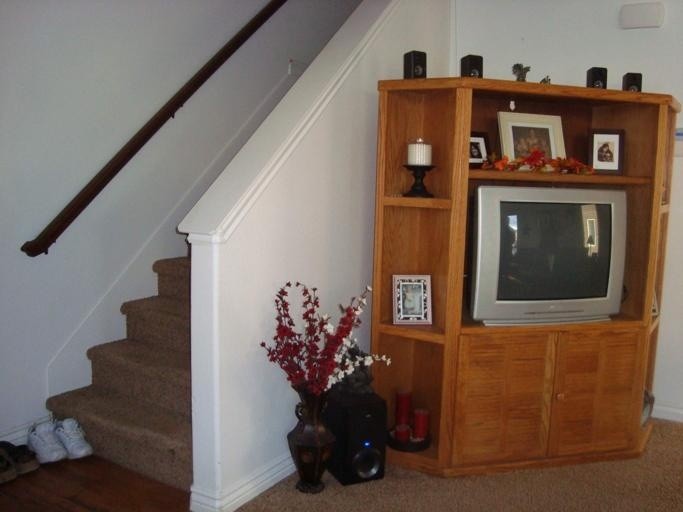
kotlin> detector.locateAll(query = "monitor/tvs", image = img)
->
[471,185,628,327]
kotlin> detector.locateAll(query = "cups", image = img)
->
[392,389,411,443]
[413,409,430,441]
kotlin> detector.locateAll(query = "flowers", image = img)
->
[481,146,599,176]
[258,280,392,394]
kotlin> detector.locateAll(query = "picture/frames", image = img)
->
[582,129,630,177]
[468,129,493,169]
[498,110,567,175]
[392,274,434,327]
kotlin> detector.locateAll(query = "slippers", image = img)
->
[0,441,40,475]
[0,447,17,484]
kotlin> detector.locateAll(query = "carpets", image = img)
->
[234,414,682,512]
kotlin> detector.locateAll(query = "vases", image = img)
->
[285,393,333,493]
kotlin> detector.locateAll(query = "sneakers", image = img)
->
[52,418,93,460]
[27,421,68,465]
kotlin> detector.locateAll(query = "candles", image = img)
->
[396,388,412,424]
[414,407,429,439]
[396,424,411,442]
[406,136,432,165]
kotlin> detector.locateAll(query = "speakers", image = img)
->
[586,67,607,89]
[403,50,426,79]
[460,55,483,78]
[622,73,642,93]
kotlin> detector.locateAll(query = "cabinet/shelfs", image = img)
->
[368,76,681,480]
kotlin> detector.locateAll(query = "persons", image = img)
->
[404,287,416,312]
[517,128,548,157]
[605,151,613,160]
[469,143,480,160]
[598,144,610,159]
